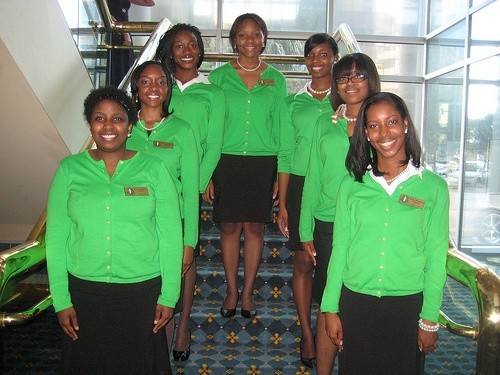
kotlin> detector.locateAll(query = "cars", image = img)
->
[449,160,485,189]
[435,159,458,176]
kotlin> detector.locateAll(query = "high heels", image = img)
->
[299,339,316,370]
[173,329,191,362]
[241,290,257,318]
[220,290,239,318]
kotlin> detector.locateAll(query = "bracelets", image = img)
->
[419,318,439,332]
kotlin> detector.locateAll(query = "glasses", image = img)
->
[335,74,368,84]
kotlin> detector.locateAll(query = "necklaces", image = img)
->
[343,109,357,121]
[137,110,165,130]
[387,168,407,182]
[308,84,331,94]
[237,57,261,71]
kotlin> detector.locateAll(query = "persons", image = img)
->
[45,87,183,375]
[104,0,155,85]
[320,92,448,375]
[207,14,288,317]
[157,23,226,193]
[299,53,381,375]
[277,33,340,369]
[131,61,199,362]
